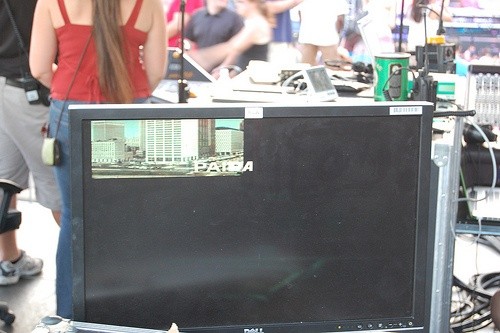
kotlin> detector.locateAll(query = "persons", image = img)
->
[29,0,167,319]
[163,0,350,81]
[407,0,482,50]
[0,0,63,285]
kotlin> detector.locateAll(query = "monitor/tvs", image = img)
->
[67,102,434,333]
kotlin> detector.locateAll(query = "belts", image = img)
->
[5,78,26,89]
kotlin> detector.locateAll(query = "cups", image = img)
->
[374,52,412,102]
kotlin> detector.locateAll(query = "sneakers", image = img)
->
[0,249,43,285]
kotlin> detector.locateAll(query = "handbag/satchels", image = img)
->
[42,137,61,166]
